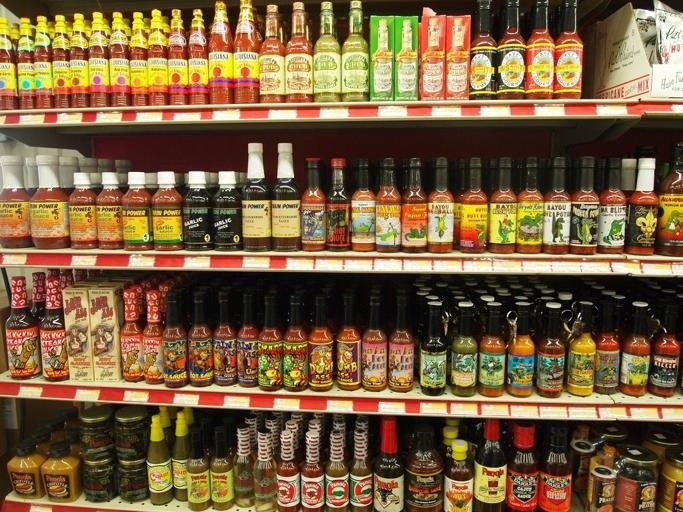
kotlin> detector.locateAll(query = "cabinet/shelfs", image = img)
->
[0,0,683,511]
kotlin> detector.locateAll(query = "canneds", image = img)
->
[116,458,148,502]
[114,406,149,461]
[78,406,114,461]
[81,455,117,503]
[573,422,683,512]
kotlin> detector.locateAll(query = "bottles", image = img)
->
[1,140,683,257]
[1,0,585,110]
[7,407,87,503]
[0,268,683,400]
[147,406,591,512]
